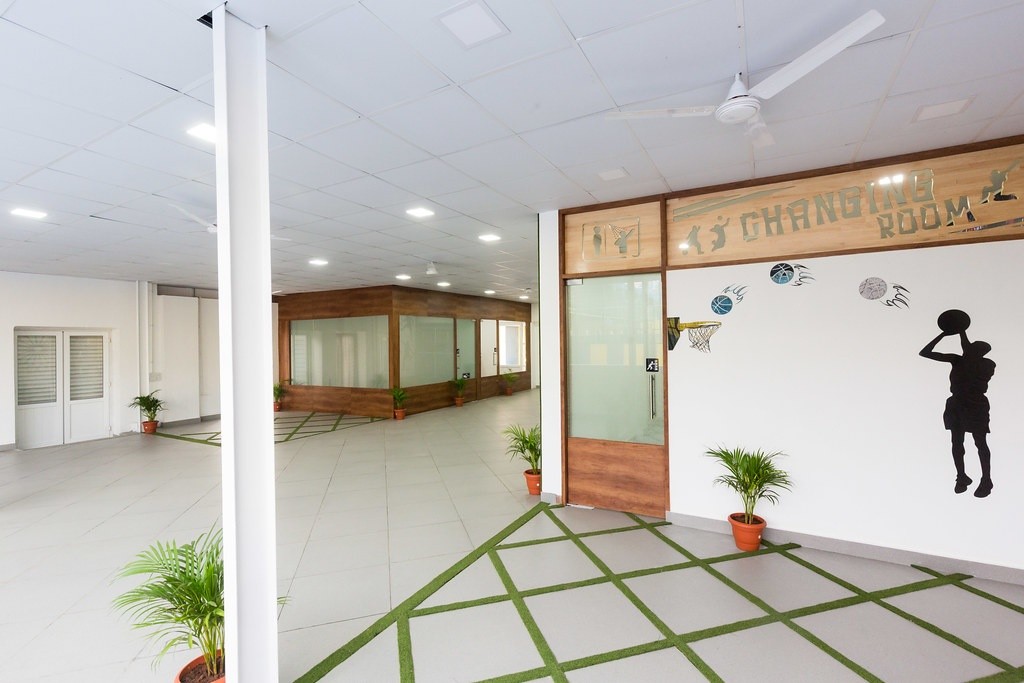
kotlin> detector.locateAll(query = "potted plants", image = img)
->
[127,389,168,435]
[112,518,291,683]
[704,443,795,552]
[391,386,408,421]
[448,377,468,407]
[273,383,289,412]
[502,367,519,395]
[504,425,541,495]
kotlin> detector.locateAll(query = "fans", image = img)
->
[174,204,292,242]
[603,9,887,148]
